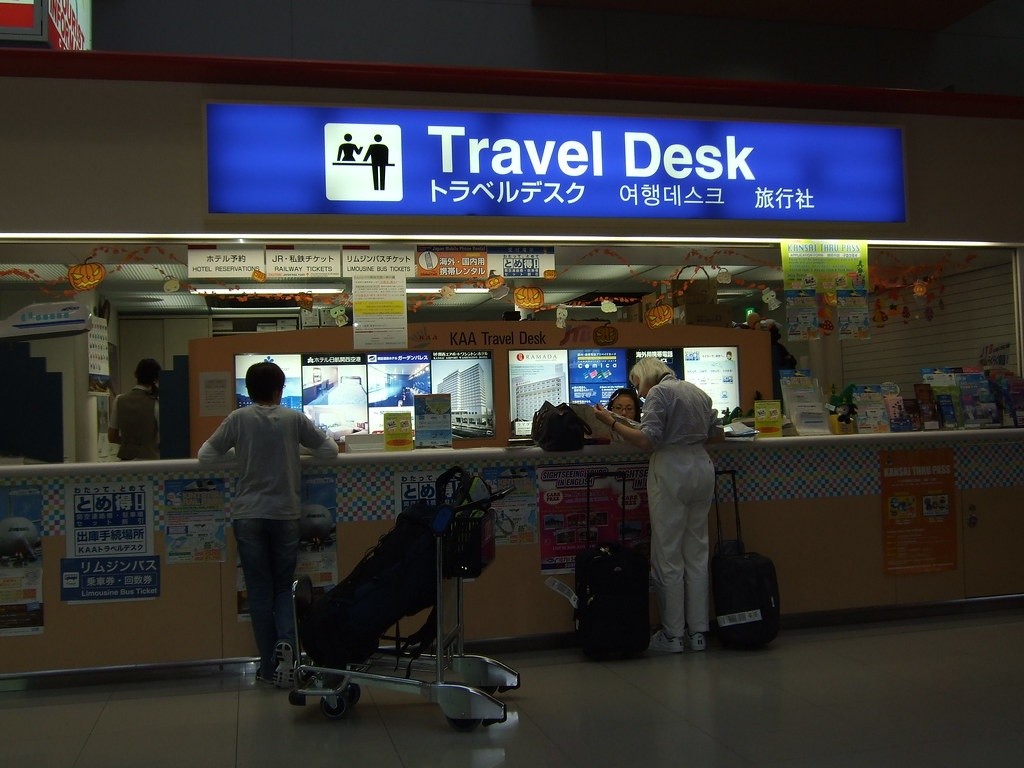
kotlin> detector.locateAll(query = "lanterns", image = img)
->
[645,304,673,330]
[593,324,619,347]
[487,275,504,290]
[68,257,106,292]
[515,287,543,309]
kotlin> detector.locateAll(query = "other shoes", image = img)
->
[273,643,295,688]
[256,668,275,684]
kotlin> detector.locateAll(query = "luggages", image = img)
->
[575,472,650,660]
[712,470,779,648]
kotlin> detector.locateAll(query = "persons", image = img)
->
[107,358,161,461]
[594,357,724,653]
[607,388,641,423]
[197,363,339,682]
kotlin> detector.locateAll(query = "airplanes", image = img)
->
[0,488,42,568]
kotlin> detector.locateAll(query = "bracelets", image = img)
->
[611,420,617,432]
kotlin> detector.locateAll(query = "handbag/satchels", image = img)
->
[671,265,717,306]
[531,401,592,451]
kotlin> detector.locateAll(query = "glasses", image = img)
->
[633,384,639,393]
[611,406,635,412]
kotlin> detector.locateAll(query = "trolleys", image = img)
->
[286,484,522,734]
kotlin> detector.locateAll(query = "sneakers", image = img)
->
[683,628,706,651]
[648,630,684,653]
